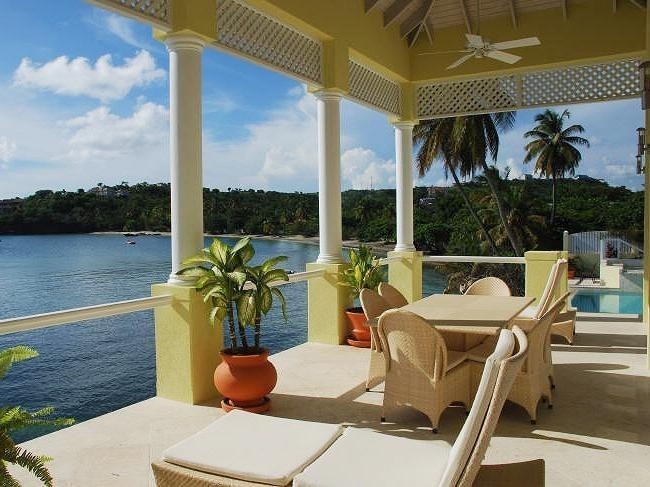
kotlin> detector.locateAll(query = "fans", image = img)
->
[417,0,541,72]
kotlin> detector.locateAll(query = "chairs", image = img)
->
[464,277,511,296]
[377,308,473,432]
[468,291,570,425]
[358,289,386,391]
[512,259,568,332]
[376,281,408,309]
[150,324,530,487]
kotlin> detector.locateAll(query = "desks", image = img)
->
[400,292,537,351]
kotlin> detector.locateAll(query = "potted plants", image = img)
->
[336,241,389,348]
[175,237,296,414]
